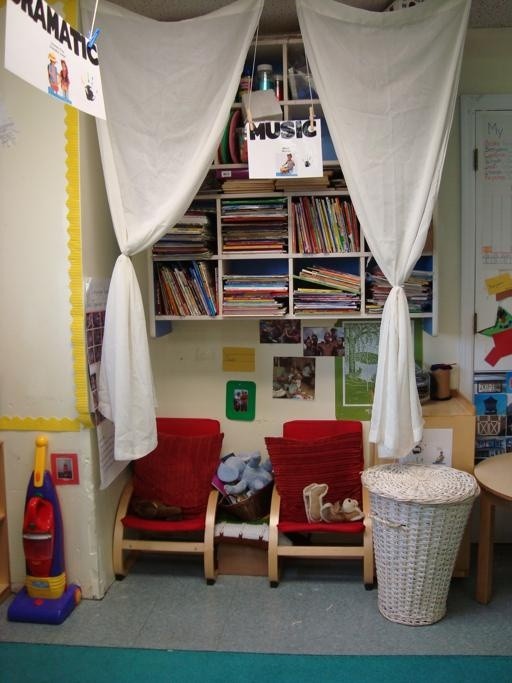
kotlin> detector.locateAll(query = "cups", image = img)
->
[429,364,452,399]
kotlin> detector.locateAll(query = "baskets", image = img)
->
[360,462,481,627]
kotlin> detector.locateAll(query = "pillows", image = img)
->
[129,430,227,512]
[264,431,364,522]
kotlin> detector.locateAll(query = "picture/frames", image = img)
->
[48,451,80,485]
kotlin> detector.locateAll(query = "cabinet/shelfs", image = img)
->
[150,192,438,338]
[371,386,477,583]
[1,441,13,605]
[209,37,342,169]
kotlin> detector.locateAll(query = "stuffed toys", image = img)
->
[216,447,275,495]
[300,480,365,524]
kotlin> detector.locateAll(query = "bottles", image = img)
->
[239,64,283,100]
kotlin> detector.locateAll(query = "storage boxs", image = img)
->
[214,521,271,576]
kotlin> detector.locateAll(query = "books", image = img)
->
[154,168,433,311]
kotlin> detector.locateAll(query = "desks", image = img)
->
[473,451,512,605]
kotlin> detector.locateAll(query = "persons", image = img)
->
[233,318,345,410]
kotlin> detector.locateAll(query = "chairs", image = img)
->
[110,416,225,588]
[267,419,378,589]
